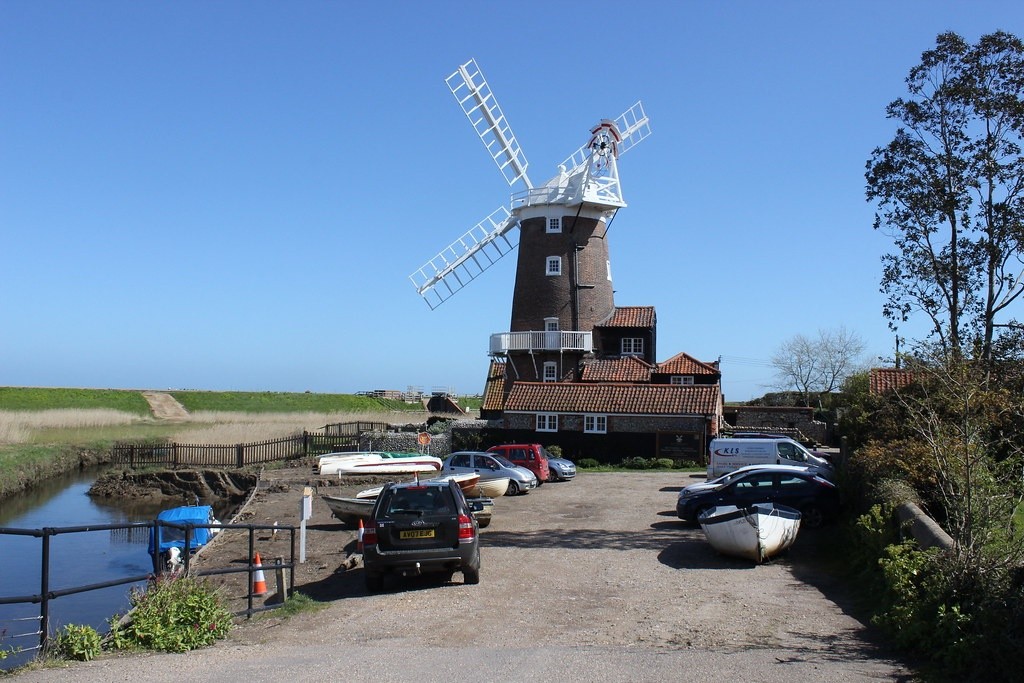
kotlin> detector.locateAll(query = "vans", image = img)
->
[475,444,551,486]
[705,436,832,482]
[731,432,834,462]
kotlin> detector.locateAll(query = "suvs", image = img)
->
[362,479,481,590]
[440,450,538,497]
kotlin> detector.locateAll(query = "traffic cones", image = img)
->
[355,518,365,553]
[247,552,274,596]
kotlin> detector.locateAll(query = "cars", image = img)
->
[676,469,844,530]
[677,465,847,498]
[529,448,577,483]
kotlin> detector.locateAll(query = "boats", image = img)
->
[147,494,222,572]
[313,450,443,476]
[696,496,804,566]
[356,470,480,496]
[321,493,495,528]
[463,477,511,498]
